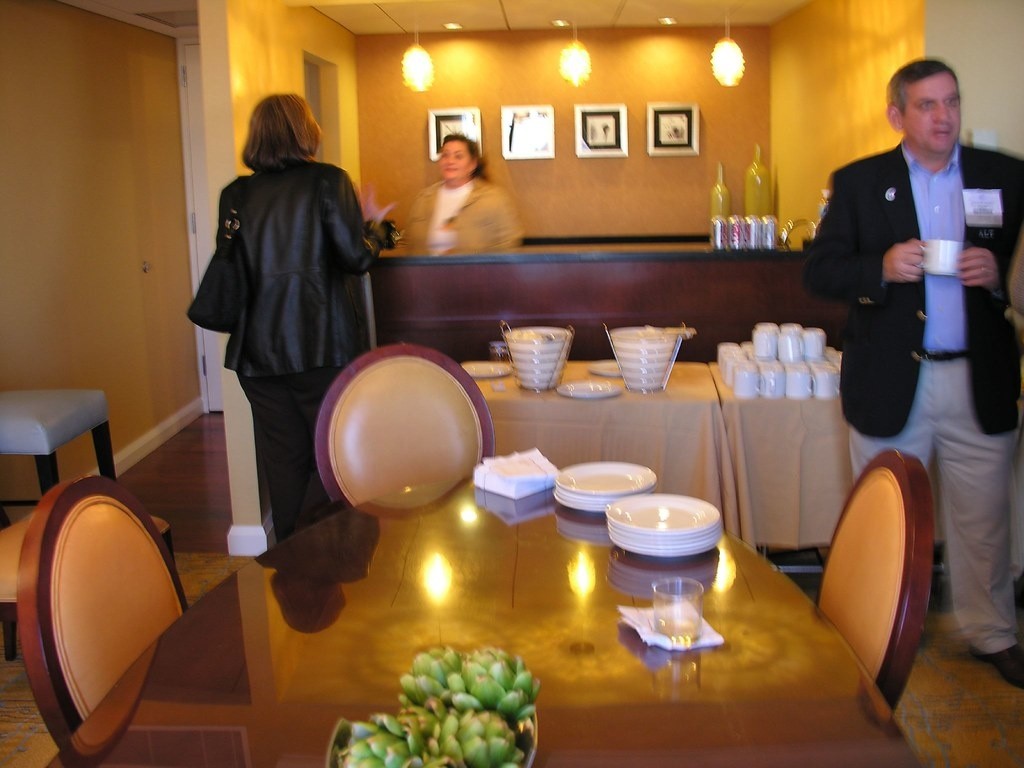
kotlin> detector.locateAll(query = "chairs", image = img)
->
[16,475,191,750]
[0,514,190,657]
[314,343,495,508]
[816,450,935,711]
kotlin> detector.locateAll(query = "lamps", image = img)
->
[558,17,591,87]
[710,8,746,87]
[401,9,435,92]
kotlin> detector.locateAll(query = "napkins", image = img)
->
[617,598,725,651]
[472,448,559,501]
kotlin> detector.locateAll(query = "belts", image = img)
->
[920,350,967,362]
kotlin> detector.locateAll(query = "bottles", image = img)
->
[816,189,830,223]
[710,160,729,217]
[743,143,774,215]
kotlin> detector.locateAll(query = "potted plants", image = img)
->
[329,644,542,768]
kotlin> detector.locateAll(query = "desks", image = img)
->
[46,477,926,768]
[709,363,1024,581]
[459,360,741,540]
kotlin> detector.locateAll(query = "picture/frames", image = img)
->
[646,101,700,157]
[500,105,556,160]
[574,104,629,158]
[428,107,483,162]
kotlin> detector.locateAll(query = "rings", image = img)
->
[982,267,995,276]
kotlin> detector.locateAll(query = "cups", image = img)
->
[489,341,508,362]
[650,576,705,645]
[716,322,842,400]
[912,239,965,277]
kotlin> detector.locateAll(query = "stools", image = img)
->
[0,389,118,529]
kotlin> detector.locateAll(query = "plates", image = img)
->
[608,545,719,599]
[553,460,659,513]
[460,361,511,378]
[586,362,622,377]
[605,492,723,558]
[556,506,611,545]
[556,380,623,399]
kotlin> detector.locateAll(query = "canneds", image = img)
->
[711,215,777,249]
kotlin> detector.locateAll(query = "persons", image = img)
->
[402,133,525,252]
[803,59,1024,689]
[216,94,402,545]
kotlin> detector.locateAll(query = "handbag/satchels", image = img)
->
[186,177,242,332]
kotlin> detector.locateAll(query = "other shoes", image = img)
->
[971,642,1024,690]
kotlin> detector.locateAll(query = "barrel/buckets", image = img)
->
[499,319,575,393]
[603,322,695,393]
